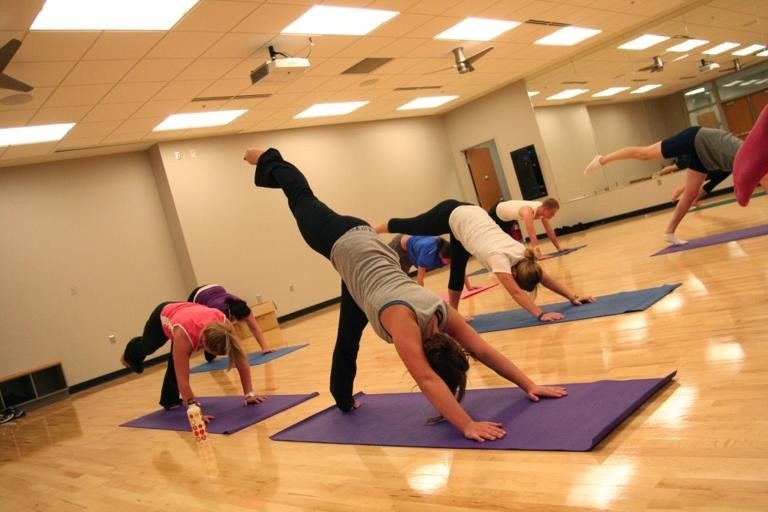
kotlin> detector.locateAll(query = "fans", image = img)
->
[718,59,747,73]
[422,46,494,77]
[638,56,665,74]
[0,38,34,94]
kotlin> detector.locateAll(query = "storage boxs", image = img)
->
[232,300,279,341]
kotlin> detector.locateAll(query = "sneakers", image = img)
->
[0,407,24,424]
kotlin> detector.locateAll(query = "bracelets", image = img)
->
[536,309,549,322]
[570,295,582,304]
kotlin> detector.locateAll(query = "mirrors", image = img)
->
[524,0,768,204]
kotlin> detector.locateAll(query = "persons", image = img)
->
[115,300,271,424]
[670,171,732,209]
[487,196,567,261]
[583,125,768,247]
[241,146,569,444]
[374,198,578,323]
[186,283,279,364]
[652,156,688,180]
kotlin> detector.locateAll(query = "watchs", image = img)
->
[243,390,258,399]
